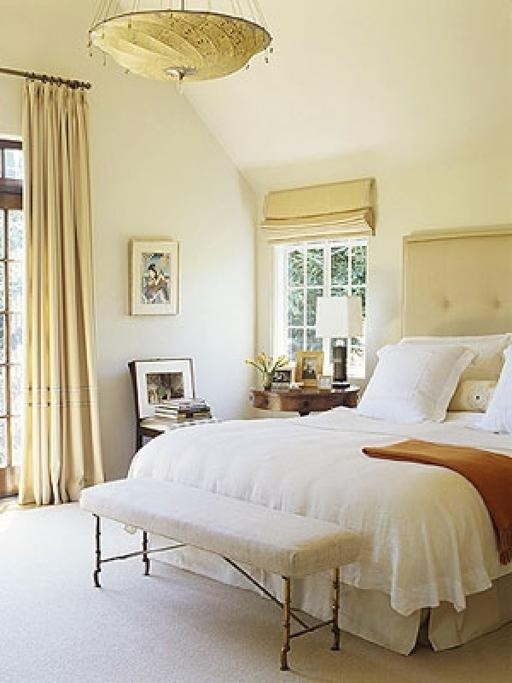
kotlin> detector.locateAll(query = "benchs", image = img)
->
[79,479,361,671]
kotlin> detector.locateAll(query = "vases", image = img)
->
[262,374,272,390]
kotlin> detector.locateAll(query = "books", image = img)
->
[153,397,212,423]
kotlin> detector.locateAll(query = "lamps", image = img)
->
[315,296,362,387]
[87,0,274,84]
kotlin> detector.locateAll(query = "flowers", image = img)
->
[245,352,289,374]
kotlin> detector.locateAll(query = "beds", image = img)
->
[127,222,512,656]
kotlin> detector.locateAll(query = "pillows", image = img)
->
[358,332,512,434]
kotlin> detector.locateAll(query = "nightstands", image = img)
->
[250,386,361,416]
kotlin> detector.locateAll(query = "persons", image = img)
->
[302,362,317,380]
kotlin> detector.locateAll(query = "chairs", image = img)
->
[127,362,219,453]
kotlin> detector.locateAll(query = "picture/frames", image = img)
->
[133,357,196,421]
[295,351,324,387]
[128,237,180,317]
